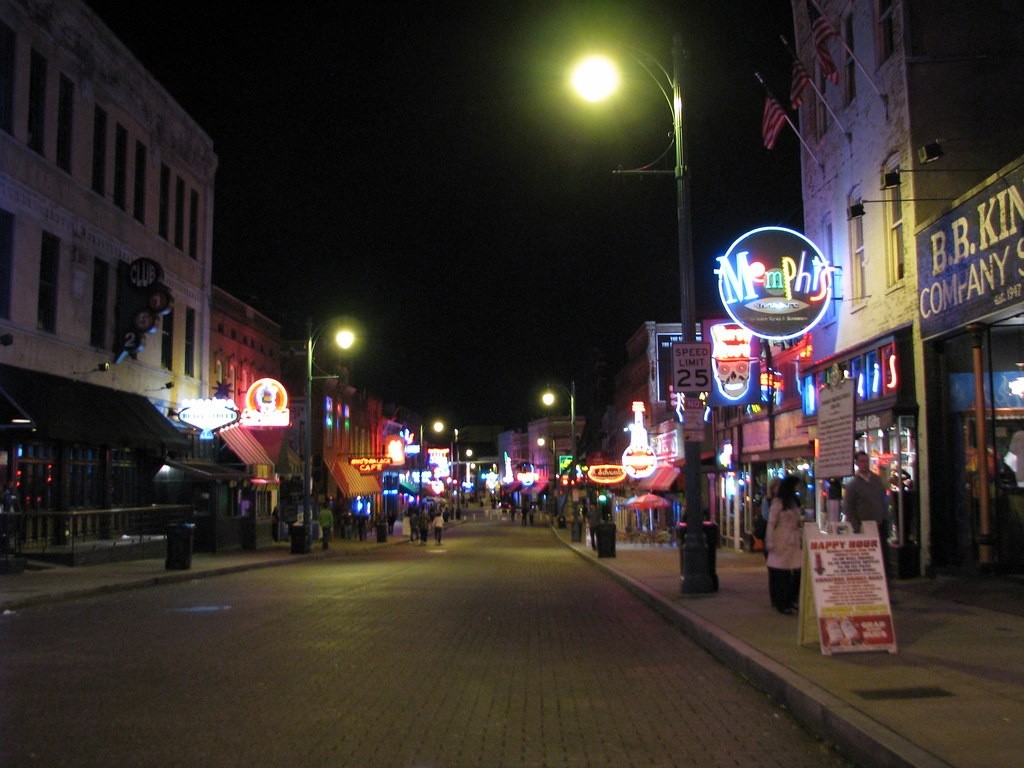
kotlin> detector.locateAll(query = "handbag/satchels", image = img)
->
[752,515,767,540]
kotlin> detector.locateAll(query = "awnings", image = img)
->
[325,460,382,497]
[638,468,680,491]
[0,364,193,452]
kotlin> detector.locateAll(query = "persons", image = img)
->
[520,503,535,526]
[845,451,893,581]
[272,506,279,542]
[409,510,419,543]
[759,474,804,615]
[417,510,430,545]
[343,511,352,541]
[357,511,366,541]
[431,513,444,544]
[585,503,599,551]
[318,504,333,551]
[387,512,396,535]
[510,504,515,522]
[0,480,17,513]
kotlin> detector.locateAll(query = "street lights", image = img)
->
[537,432,557,516]
[569,29,716,594]
[542,380,581,543]
[304,316,355,553]
[419,421,444,511]
[455,449,473,520]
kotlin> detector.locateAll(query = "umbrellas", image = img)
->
[628,492,669,530]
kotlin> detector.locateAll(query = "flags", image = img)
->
[762,88,787,151]
[788,45,810,110]
[805,0,839,85]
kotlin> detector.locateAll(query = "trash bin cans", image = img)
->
[571,520,583,541]
[165,523,195,570]
[376,523,388,543]
[289,525,309,554]
[595,523,616,558]
[678,522,719,590]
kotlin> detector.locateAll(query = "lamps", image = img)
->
[74,364,109,374]
[847,197,958,221]
[0,334,13,345]
[146,382,174,391]
[880,168,999,190]
[918,137,1024,163]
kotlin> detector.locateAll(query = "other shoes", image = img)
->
[780,607,793,615]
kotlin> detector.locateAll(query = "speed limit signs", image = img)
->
[671,341,712,394]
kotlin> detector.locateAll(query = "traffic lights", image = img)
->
[598,492,607,505]
